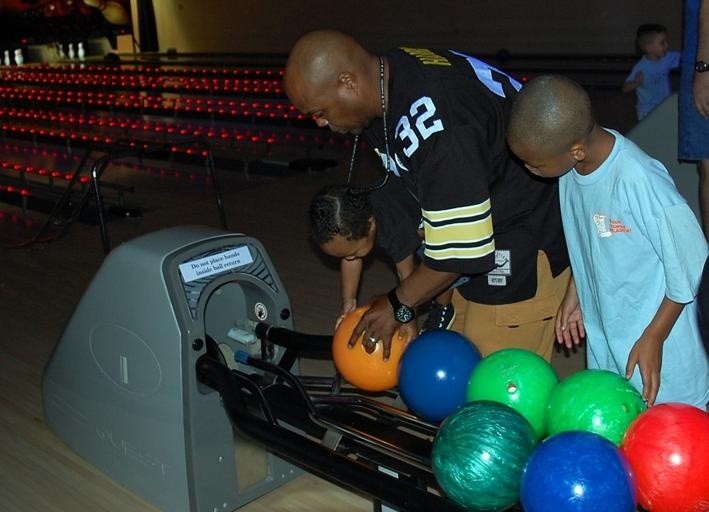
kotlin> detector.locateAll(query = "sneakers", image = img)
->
[417,299,456,337]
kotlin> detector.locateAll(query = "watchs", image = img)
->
[694,59,709,73]
[388,288,415,323]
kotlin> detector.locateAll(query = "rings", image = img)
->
[368,335,379,346]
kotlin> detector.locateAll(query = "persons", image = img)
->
[679,0,709,244]
[505,72,709,416]
[621,25,681,122]
[282,29,571,367]
[309,177,457,335]
